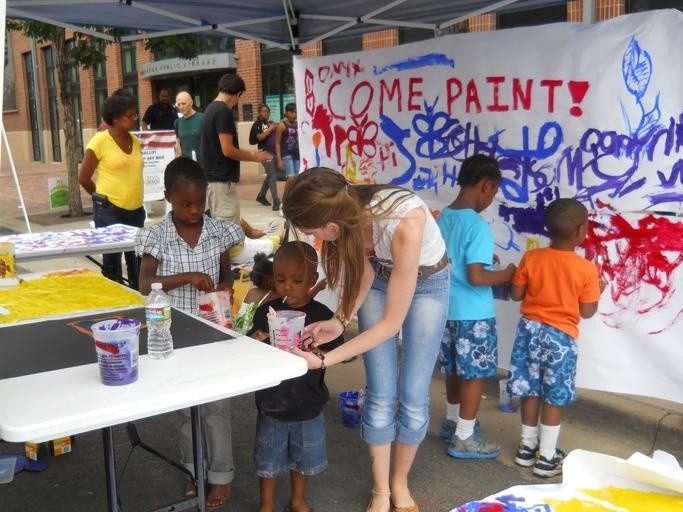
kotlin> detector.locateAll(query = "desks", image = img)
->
[0,266,308,512]
[0,222,144,286]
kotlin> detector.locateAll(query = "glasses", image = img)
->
[120,113,140,119]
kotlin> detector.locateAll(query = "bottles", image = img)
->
[145,282,173,359]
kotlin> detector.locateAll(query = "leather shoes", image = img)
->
[271,198,281,211]
[393,486,419,512]
[365,489,391,512]
[256,193,269,209]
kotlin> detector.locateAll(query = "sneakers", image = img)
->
[440,418,486,443]
[447,425,502,459]
[515,438,540,470]
[531,448,568,478]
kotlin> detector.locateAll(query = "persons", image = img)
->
[274,103,300,177]
[141,88,177,130]
[434,154,516,459]
[246,241,356,511]
[204,208,278,239]
[249,105,283,211]
[198,73,274,226]
[283,166,450,512]
[173,92,204,162]
[98,88,135,130]
[233,252,328,337]
[78,94,146,292]
[510,197,601,478]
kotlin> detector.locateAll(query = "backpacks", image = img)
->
[135,155,245,507]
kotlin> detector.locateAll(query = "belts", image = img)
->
[370,253,450,285]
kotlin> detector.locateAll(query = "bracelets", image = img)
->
[333,312,350,330]
[312,349,328,371]
